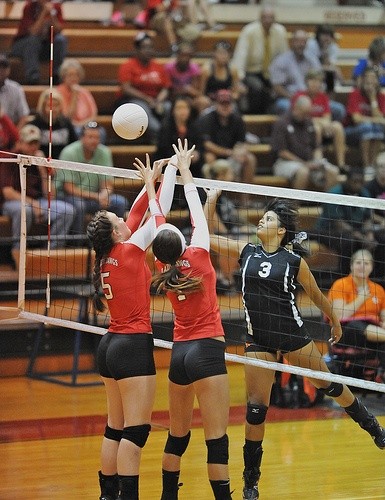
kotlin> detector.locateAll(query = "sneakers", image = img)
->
[363,416,385,450]
[243,470,260,500]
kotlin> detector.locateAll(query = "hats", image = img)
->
[19,124,41,144]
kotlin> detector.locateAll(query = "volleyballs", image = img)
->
[111,103,149,140]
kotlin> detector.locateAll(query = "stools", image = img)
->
[26,283,105,388]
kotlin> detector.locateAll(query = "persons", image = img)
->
[86,143,196,500]
[0,0,385,275]
[203,185,385,500]
[324,249,385,353]
[133,138,238,500]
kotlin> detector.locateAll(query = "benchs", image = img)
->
[0,0,385,361]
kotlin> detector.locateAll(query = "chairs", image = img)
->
[318,313,385,396]
